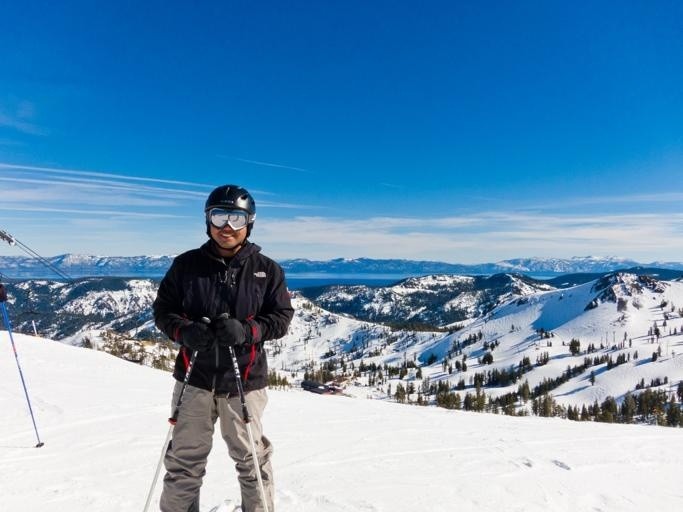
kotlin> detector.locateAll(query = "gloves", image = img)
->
[223,319,261,348]
[176,321,213,351]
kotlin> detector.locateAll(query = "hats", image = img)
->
[205,184,255,236]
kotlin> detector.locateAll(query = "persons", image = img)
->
[151,183,295,511]
[0,285,10,303]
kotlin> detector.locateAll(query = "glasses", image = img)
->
[205,209,256,233]
